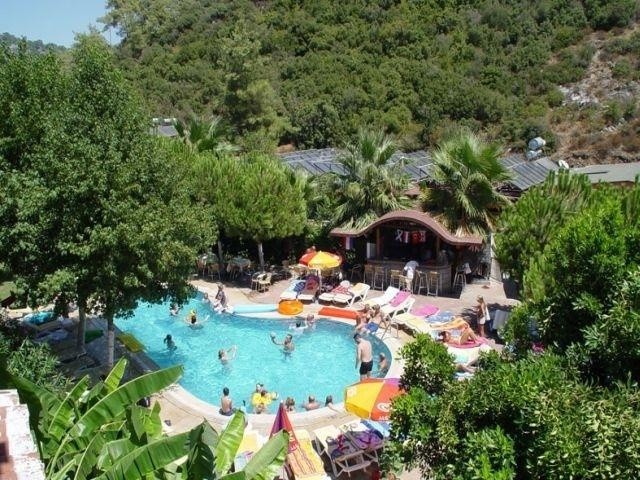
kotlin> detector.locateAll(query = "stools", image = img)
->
[453,261,467,288]
[362,265,440,298]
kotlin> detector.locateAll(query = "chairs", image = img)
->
[370,286,416,318]
[319,280,370,306]
[234,417,391,480]
[392,303,469,342]
[13,309,95,377]
[196,250,272,293]
[280,268,320,303]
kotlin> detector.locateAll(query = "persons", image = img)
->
[402,257,420,293]
[248,381,335,416]
[289,314,320,330]
[435,247,475,284]
[270,330,297,355]
[452,360,476,374]
[168,281,230,330]
[98,374,106,383]
[306,273,340,292]
[492,319,494,320]
[217,387,238,416]
[347,303,391,383]
[164,333,178,356]
[216,344,237,365]
[436,295,493,347]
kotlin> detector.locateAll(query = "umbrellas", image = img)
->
[269,400,300,454]
[345,377,412,442]
